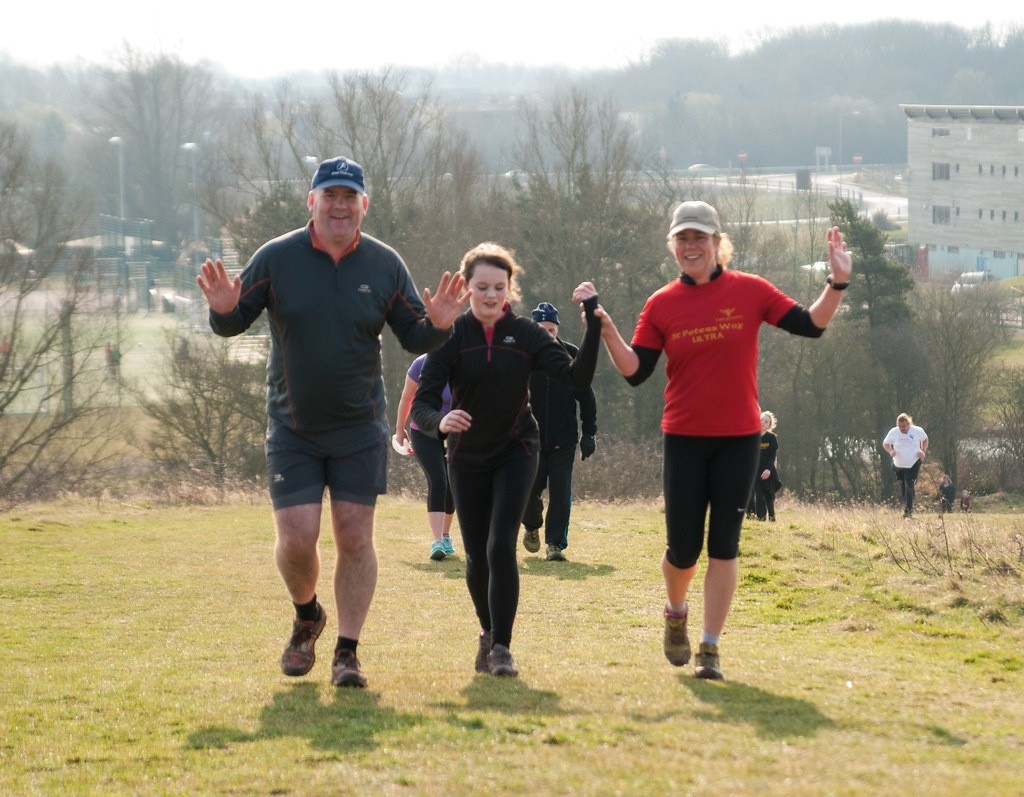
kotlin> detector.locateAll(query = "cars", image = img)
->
[687,164,718,176]
[947,271,994,300]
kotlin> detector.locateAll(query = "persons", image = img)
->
[395,353,455,563]
[197,154,473,687]
[580,200,854,680]
[521,301,597,562]
[960,489,973,513]
[883,413,929,519]
[747,410,782,522]
[939,474,956,514]
[411,241,603,678]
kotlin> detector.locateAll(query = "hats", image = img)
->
[531,301,560,326]
[667,201,721,238]
[311,156,365,195]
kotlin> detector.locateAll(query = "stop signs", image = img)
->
[739,151,748,161]
[852,154,863,165]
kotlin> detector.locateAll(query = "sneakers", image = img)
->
[695,641,723,678]
[443,536,454,553]
[663,603,691,667]
[489,644,518,677]
[546,544,567,561]
[331,649,368,687]
[475,630,491,674]
[279,604,327,677]
[430,540,446,559]
[523,529,541,553]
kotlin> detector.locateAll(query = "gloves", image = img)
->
[580,435,596,461]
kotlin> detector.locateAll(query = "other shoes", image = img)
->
[905,517,911,522]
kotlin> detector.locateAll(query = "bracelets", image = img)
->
[827,273,849,291]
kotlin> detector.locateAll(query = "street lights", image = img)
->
[179,142,201,276]
[107,136,126,260]
[839,111,860,178]
